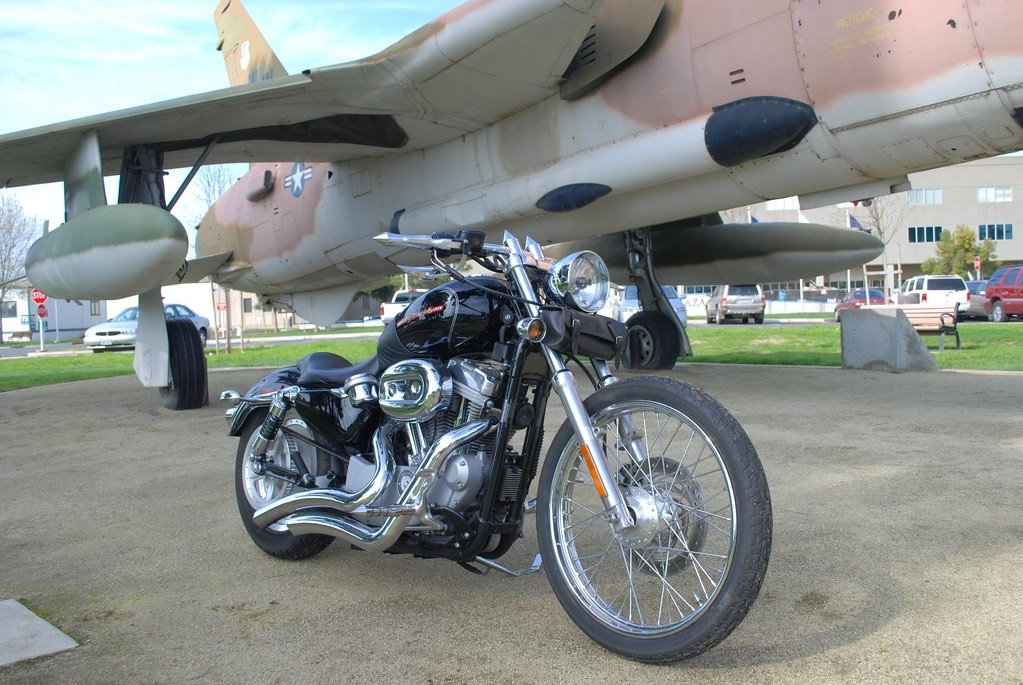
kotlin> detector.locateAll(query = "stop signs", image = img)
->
[31,287,47,304]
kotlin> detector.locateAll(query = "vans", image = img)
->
[897,274,970,322]
[963,279,998,322]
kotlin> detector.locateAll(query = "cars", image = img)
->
[834,289,895,323]
[613,283,686,326]
[83,304,211,353]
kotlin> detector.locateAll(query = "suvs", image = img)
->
[705,283,766,324]
[982,265,1023,322]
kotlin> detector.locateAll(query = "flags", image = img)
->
[849,214,872,234]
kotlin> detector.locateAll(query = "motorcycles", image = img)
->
[219,227,773,666]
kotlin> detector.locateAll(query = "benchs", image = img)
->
[859,302,960,353]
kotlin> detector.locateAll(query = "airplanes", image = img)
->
[0,0,1023,411]
